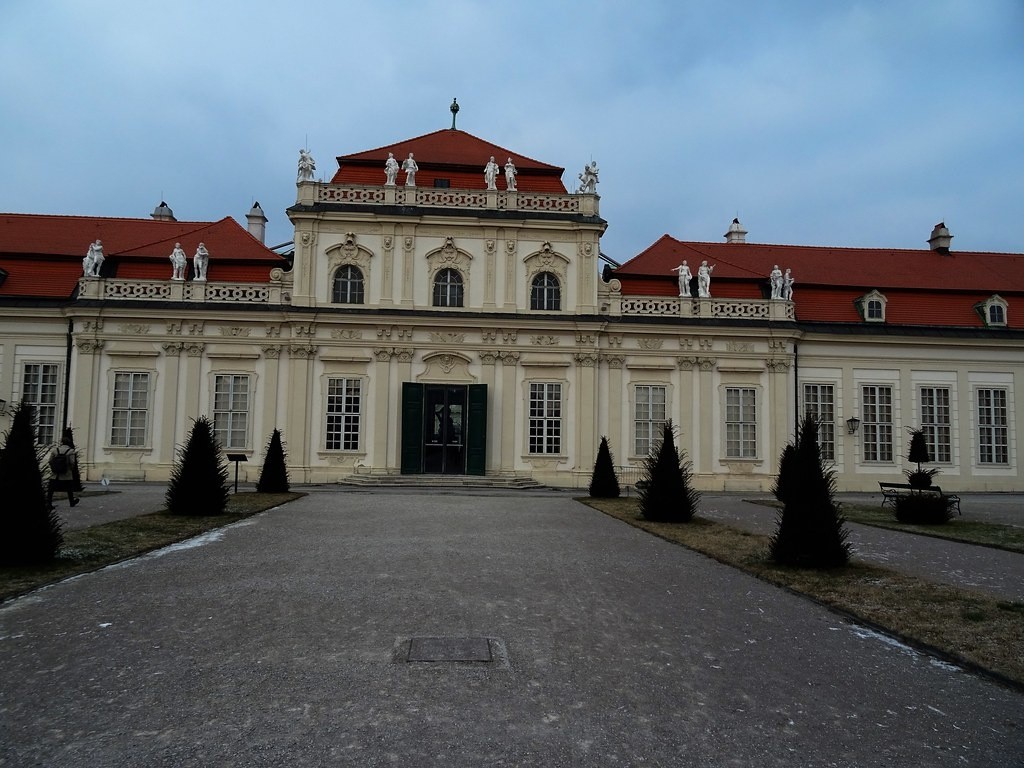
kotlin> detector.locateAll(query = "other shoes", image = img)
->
[70,498,79,507]
[52,506,55,510]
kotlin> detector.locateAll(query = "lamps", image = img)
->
[0,399,6,416]
[846,416,860,434]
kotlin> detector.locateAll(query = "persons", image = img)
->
[504,157,517,189]
[698,260,713,297]
[670,260,692,294]
[401,153,418,186]
[770,265,795,300]
[297,149,315,178]
[88,239,106,277]
[48,436,81,507]
[384,152,400,184]
[193,243,209,280]
[171,243,188,279]
[579,162,599,192]
[484,156,500,189]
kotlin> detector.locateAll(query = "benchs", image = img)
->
[878,481,962,516]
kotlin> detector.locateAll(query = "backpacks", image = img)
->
[48,448,70,476]
